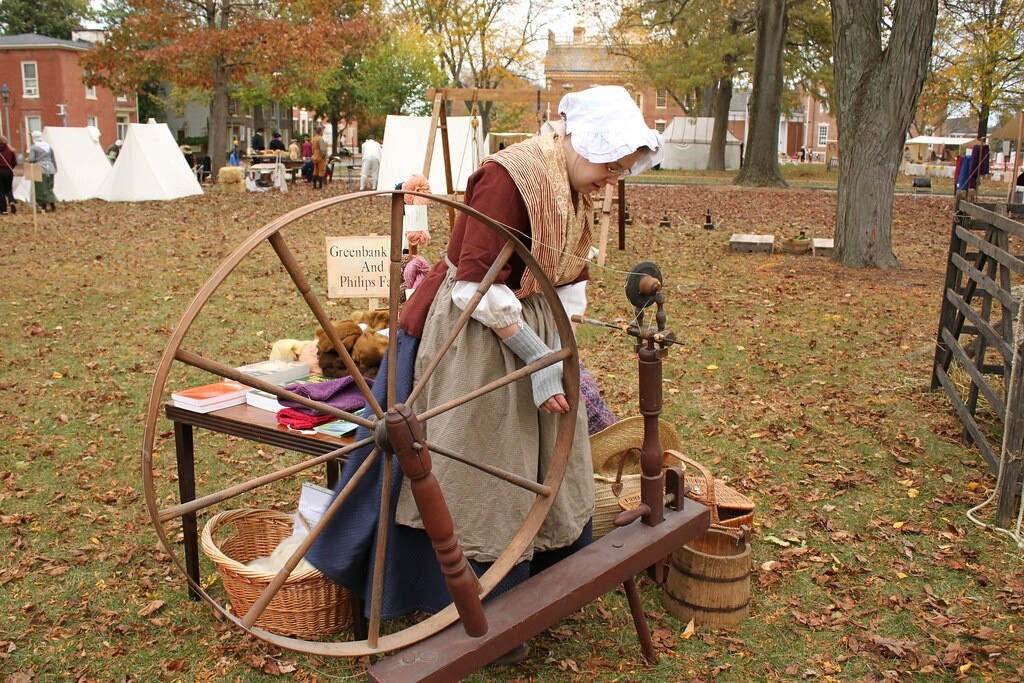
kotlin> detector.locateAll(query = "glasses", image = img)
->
[604,160,633,182]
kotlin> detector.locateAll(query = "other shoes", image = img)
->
[10,202,17,214]
[2,212,8,214]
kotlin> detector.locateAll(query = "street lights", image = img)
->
[0,83,11,145]
[272,66,283,134]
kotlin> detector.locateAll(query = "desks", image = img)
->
[261,158,307,178]
[163,361,357,601]
[346,165,362,189]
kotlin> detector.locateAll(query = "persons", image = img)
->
[252,128,265,164]
[302,86,666,662]
[301,136,313,182]
[904,147,912,161]
[229,140,239,166]
[800,146,806,162]
[107,139,123,163]
[0,135,19,214]
[270,132,285,163]
[27,130,57,212]
[288,139,299,160]
[311,125,329,189]
[360,135,381,190]
[179,145,194,168]
[808,146,813,163]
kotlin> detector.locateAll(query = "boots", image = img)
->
[319,176,323,189]
[312,175,318,189]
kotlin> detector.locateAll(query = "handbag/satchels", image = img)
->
[590,444,643,541]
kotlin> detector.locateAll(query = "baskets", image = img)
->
[202,507,352,640]
[618,449,756,539]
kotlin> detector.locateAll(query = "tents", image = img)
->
[903,135,981,178]
[660,115,742,170]
[12,126,111,202]
[377,114,489,196]
[97,116,205,201]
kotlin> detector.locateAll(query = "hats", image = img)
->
[180,145,192,154]
[256,128,264,132]
[115,140,123,146]
[589,414,683,480]
[271,131,281,138]
[233,140,238,146]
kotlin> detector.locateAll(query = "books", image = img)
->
[171,381,254,414]
[246,374,337,414]
[234,359,309,385]
[313,419,359,439]
